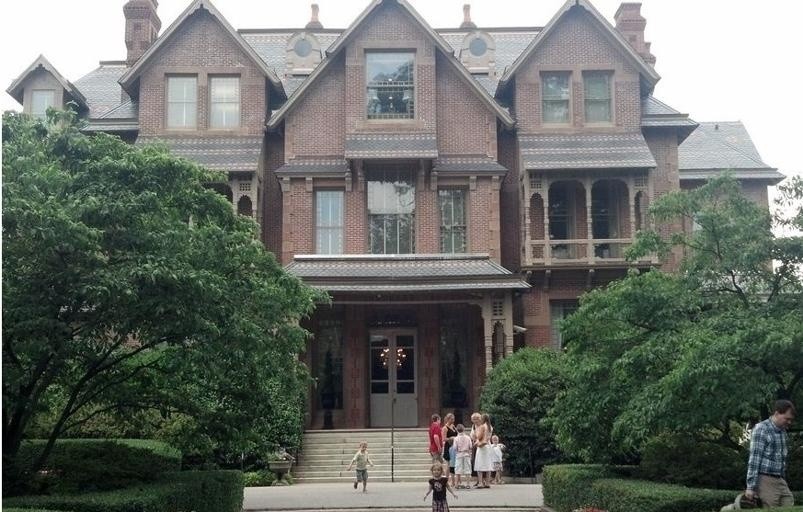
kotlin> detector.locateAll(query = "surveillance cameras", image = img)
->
[513,324,528,336]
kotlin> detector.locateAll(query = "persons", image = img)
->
[428,411,507,488]
[423,463,458,512]
[745,399,794,508]
[346,440,374,493]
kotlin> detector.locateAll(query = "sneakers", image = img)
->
[354,482,357,489]
[455,480,490,489]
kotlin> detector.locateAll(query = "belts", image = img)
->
[759,472,783,478]
